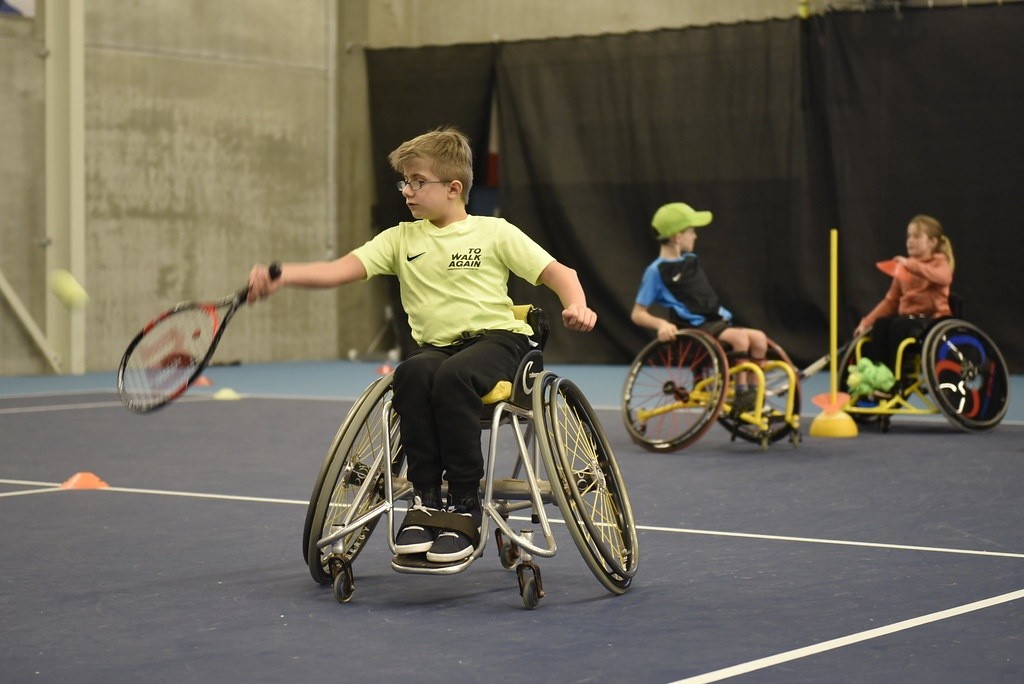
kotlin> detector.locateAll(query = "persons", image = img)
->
[245,130,596,564]
[631,202,779,416]
[849,216,952,419]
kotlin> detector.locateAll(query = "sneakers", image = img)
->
[426,497,482,563]
[394,496,446,553]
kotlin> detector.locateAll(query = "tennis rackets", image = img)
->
[116,259,284,416]
[764,337,854,397]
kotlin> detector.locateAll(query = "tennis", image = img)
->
[45,266,96,315]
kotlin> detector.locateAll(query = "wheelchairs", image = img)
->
[620,309,803,454]
[302,304,641,612]
[834,289,1014,432]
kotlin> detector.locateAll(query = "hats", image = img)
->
[651,202,713,239]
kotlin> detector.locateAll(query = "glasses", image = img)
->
[397,179,453,192]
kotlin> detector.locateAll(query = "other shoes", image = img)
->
[732,391,771,416]
